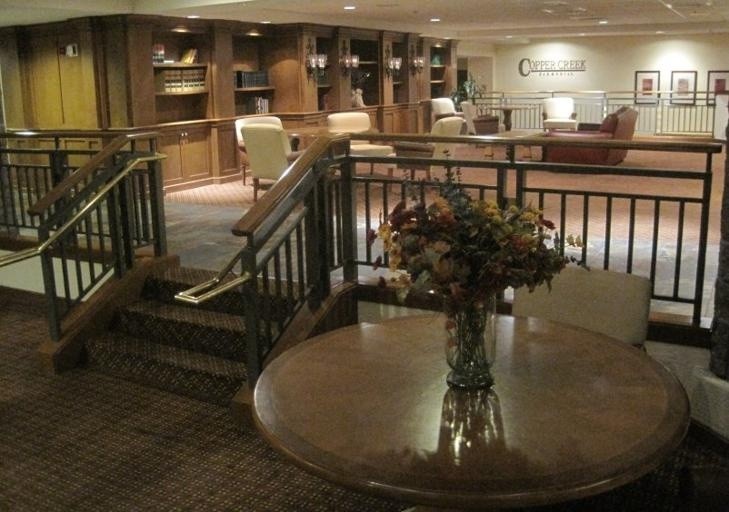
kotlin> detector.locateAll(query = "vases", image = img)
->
[446,287,497,387]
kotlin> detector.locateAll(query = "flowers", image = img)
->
[367,149,592,385]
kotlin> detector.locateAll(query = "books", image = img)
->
[246,96,269,114]
[153,43,176,64]
[180,45,200,63]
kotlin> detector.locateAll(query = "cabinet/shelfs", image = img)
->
[129,14,460,192]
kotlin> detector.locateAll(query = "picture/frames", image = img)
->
[634,69,729,107]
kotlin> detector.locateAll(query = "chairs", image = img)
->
[511,266,651,351]
[461,98,639,166]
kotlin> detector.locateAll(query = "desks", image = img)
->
[255,309,695,511]
